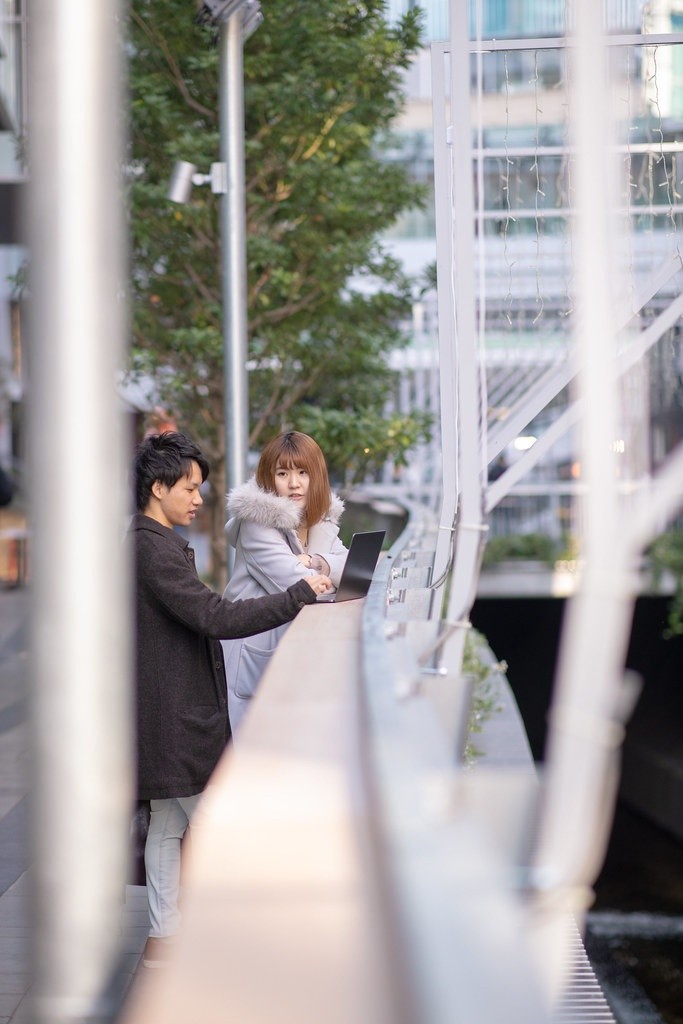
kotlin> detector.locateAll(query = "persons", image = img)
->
[220,431,351,737]
[121,429,333,970]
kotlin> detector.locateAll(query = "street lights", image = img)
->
[160,158,246,580]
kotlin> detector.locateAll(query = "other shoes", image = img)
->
[143,935,182,973]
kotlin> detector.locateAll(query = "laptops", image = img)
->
[315,529,386,603]
